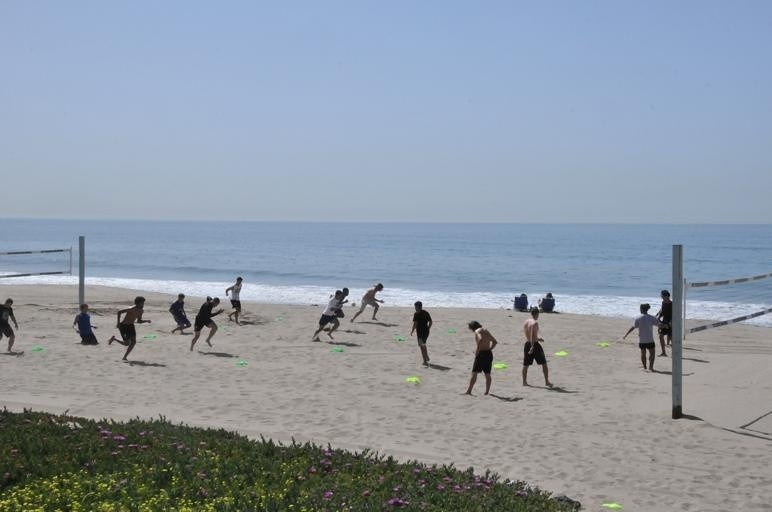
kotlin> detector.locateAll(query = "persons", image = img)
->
[191,296,226,352]
[459,320,497,396]
[169,293,192,335]
[410,300,433,368]
[1,297,21,356]
[521,308,554,386]
[108,296,151,361]
[311,289,344,343]
[621,302,666,374]
[654,290,671,357]
[225,277,244,326]
[539,292,554,309]
[329,287,350,318]
[71,303,99,344]
[518,293,528,313]
[351,283,385,323]
